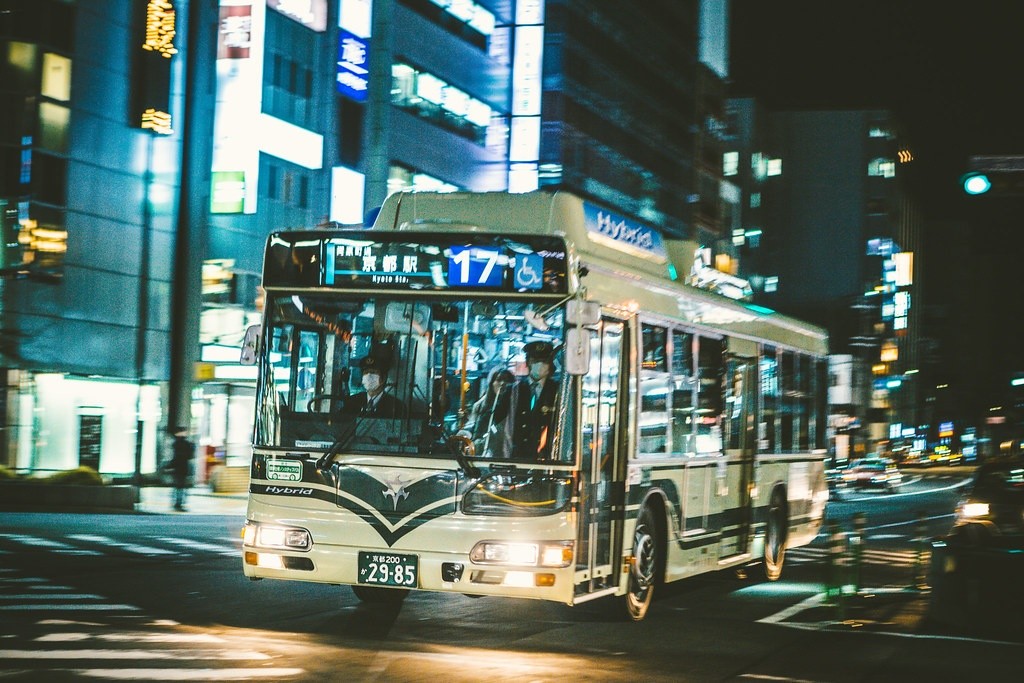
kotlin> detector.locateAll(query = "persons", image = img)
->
[168,426,195,511]
[457,341,559,461]
[336,356,408,417]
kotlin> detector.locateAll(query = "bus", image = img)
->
[239,187,834,621]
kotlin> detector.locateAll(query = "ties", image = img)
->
[369,399,373,408]
[531,382,538,411]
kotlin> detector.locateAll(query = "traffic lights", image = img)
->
[951,160,995,202]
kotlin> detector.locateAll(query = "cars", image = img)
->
[823,453,851,484]
[839,456,903,495]
[924,456,1024,605]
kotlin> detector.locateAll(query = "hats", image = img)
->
[359,356,392,371]
[522,341,554,358]
